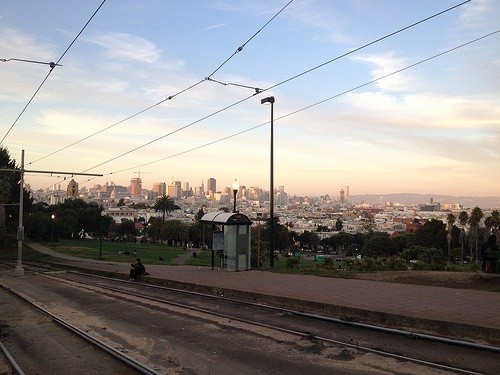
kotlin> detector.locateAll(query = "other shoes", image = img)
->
[129,278,132,281]
[132,279,135,282]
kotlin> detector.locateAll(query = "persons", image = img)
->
[313,255,321,270]
[206,244,208,250]
[133,249,137,257]
[130,258,143,282]
[123,233,127,239]
[337,259,346,269]
[325,264,337,273]
[273,251,279,267]
